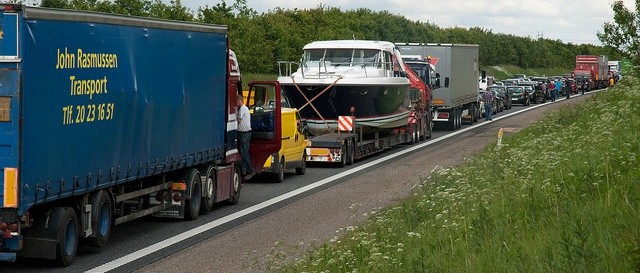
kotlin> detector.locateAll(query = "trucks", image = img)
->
[303,55,441,168]
[0,2,281,268]
[394,42,484,131]
[574,55,609,91]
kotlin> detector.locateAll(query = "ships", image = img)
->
[278,40,411,132]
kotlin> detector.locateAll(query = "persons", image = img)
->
[581,76,586,95]
[564,78,571,98]
[610,76,614,89]
[556,78,563,100]
[549,80,556,102]
[541,80,547,103]
[237,94,257,181]
[483,87,494,120]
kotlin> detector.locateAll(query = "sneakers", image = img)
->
[244,167,256,180]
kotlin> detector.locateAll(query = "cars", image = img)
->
[531,77,552,100]
[549,76,567,97]
[507,86,532,106]
[477,91,496,118]
[486,86,511,112]
[521,81,546,104]
[561,74,578,94]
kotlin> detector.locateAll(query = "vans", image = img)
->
[249,108,311,183]
